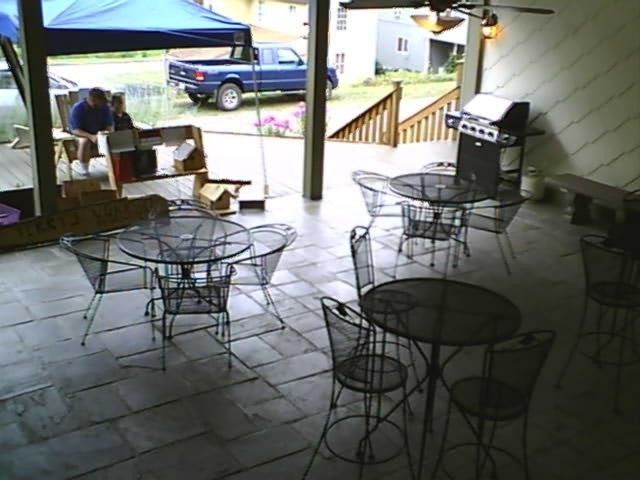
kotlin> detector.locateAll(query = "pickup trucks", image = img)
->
[167,44,339,111]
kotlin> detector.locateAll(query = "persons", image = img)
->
[68,88,115,177]
[110,95,135,131]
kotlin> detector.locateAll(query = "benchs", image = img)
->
[56,136,103,182]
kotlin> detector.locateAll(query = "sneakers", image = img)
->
[76,169,91,178]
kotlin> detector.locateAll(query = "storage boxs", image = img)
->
[1,202,21,227]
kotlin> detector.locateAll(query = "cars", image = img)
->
[0,69,82,142]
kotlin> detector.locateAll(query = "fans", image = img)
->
[340,0,556,15]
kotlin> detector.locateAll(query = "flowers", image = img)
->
[255,116,292,132]
[291,101,328,115]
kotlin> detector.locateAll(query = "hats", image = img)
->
[88,87,112,104]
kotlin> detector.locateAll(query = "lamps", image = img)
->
[410,1,465,34]
[478,11,500,40]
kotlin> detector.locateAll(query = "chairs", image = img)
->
[351,161,530,280]
[573,188,640,417]
